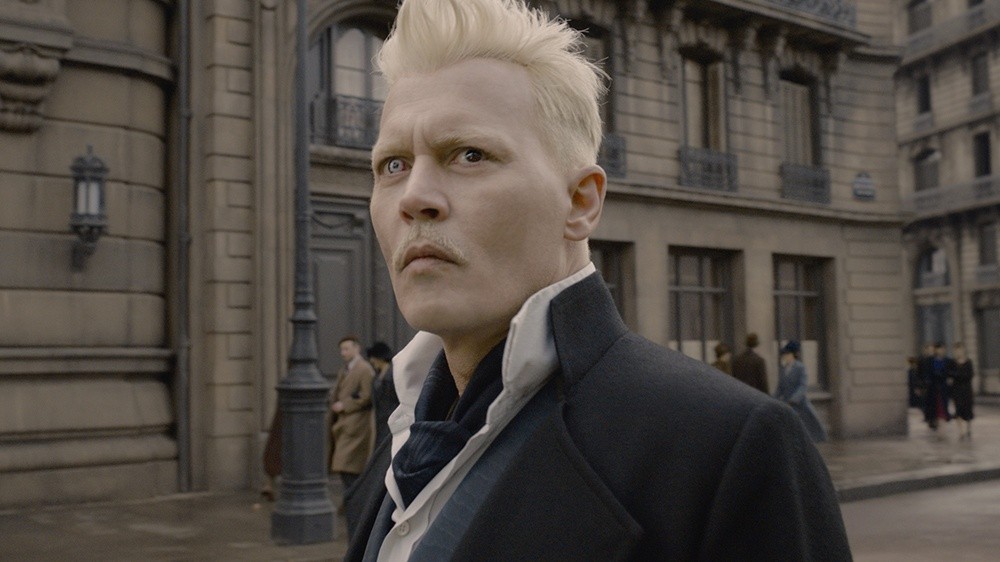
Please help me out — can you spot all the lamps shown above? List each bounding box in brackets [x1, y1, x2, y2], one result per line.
[70, 141, 110, 268]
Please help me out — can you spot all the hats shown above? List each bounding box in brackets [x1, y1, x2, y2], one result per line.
[366, 342, 392, 363]
[779, 341, 800, 357]
[714, 343, 730, 358]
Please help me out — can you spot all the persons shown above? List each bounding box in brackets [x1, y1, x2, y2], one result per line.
[775, 342, 826, 440]
[345, 1, 853, 562]
[733, 333, 768, 394]
[328, 337, 376, 488]
[712, 344, 733, 376]
[352, 342, 399, 450]
[909, 341, 974, 443]
